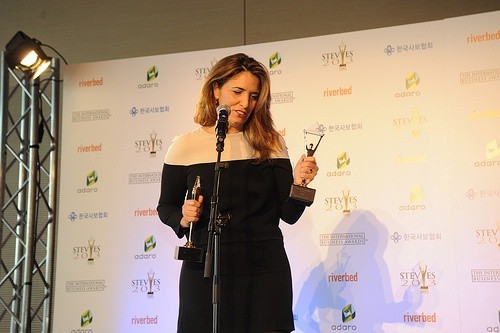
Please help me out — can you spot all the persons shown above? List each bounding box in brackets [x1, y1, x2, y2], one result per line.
[157, 52, 320, 333]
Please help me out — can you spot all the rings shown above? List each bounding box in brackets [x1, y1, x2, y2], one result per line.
[308, 168, 312, 174]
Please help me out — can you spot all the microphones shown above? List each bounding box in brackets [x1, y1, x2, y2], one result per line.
[216, 104, 231, 141]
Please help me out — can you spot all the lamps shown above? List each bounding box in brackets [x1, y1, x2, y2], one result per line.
[5, 31, 52, 80]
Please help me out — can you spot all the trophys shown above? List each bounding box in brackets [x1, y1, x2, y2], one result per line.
[173, 176, 203, 263]
[289, 129, 325, 207]
